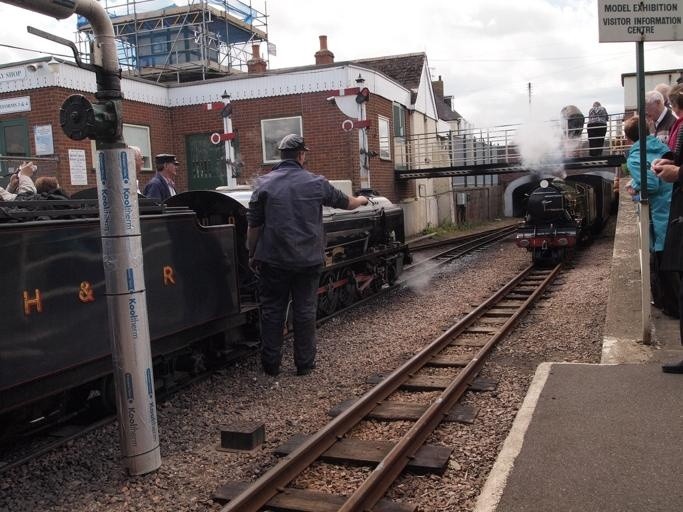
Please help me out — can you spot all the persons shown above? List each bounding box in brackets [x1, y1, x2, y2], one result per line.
[143, 154, 180, 204]
[35, 176, 60, 194]
[127, 145, 144, 194]
[246, 133, 368, 376]
[587, 102, 609, 157]
[623, 83, 682, 373]
[0, 160, 38, 202]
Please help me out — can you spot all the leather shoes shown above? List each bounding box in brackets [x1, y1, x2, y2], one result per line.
[297, 361, 317, 375]
[662, 359, 683, 374]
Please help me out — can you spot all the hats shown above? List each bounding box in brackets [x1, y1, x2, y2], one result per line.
[155, 154, 180, 164]
[277, 134, 310, 151]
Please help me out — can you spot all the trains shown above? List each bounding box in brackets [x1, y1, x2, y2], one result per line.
[512, 170, 616, 269]
[0, 176, 416, 448]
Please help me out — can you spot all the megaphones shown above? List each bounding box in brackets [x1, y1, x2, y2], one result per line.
[26, 63, 40, 73]
